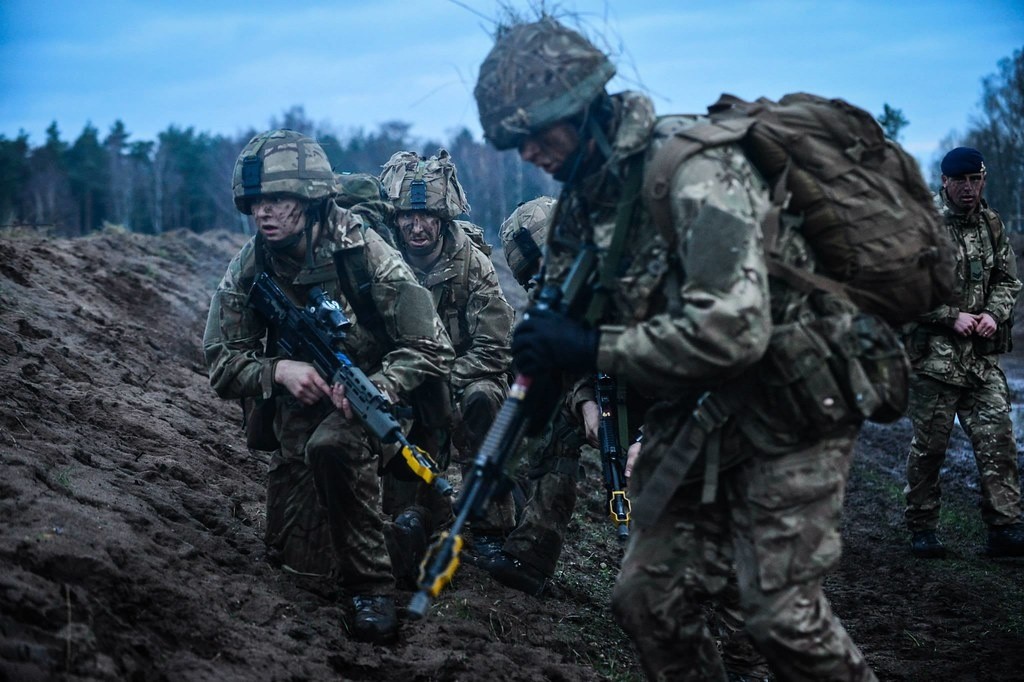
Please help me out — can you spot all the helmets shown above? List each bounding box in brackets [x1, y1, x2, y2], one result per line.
[500, 196, 557, 285]
[380, 151, 471, 222]
[232, 129, 338, 215]
[475, 17, 616, 152]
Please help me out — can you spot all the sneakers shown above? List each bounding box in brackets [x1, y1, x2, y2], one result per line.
[347, 595, 400, 644]
[391, 505, 433, 592]
[407, 492, 456, 526]
[471, 531, 502, 557]
[912, 529, 962, 559]
[988, 522, 1024, 558]
[475, 554, 546, 596]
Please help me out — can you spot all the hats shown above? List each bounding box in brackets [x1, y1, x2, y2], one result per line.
[941, 146, 986, 177]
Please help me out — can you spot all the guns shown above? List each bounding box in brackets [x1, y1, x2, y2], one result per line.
[247, 268, 461, 499]
[596, 371, 634, 543]
[404, 247, 608, 619]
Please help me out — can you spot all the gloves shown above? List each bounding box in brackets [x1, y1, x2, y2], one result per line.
[510, 308, 599, 379]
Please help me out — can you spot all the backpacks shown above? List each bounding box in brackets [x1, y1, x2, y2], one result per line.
[644, 93, 962, 327]
[243, 173, 398, 452]
[448, 219, 494, 350]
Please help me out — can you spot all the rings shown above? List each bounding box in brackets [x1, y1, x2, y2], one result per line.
[984, 332, 988, 336]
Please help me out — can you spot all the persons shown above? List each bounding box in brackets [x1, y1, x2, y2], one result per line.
[200, 129, 648, 642]
[474, 17, 877, 682]
[905, 147, 1024, 560]
